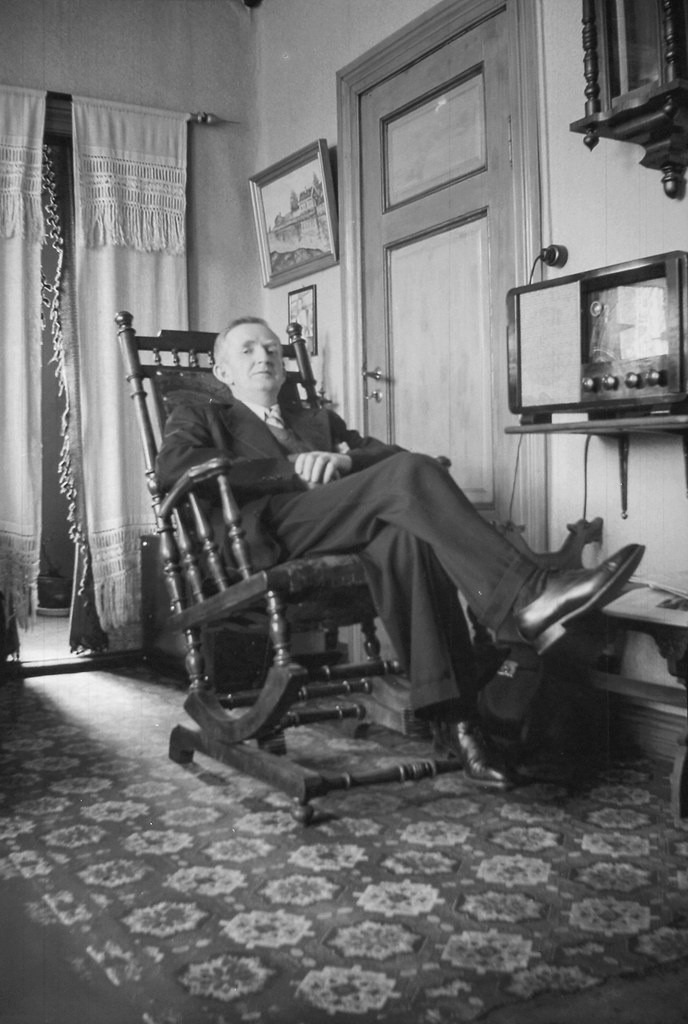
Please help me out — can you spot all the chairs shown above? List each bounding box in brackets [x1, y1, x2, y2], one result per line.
[113, 307, 460, 822]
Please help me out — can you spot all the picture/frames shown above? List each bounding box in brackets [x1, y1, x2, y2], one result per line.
[286, 282, 316, 354]
[246, 133, 338, 292]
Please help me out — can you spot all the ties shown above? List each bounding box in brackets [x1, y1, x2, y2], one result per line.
[265, 406, 287, 430]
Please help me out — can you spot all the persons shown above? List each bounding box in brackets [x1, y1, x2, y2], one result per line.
[155, 316, 645, 787]
[294, 298, 314, 353]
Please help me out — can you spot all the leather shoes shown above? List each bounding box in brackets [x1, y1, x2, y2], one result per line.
[511, 543, 645, 657]
[428, 706, 520, 789]
